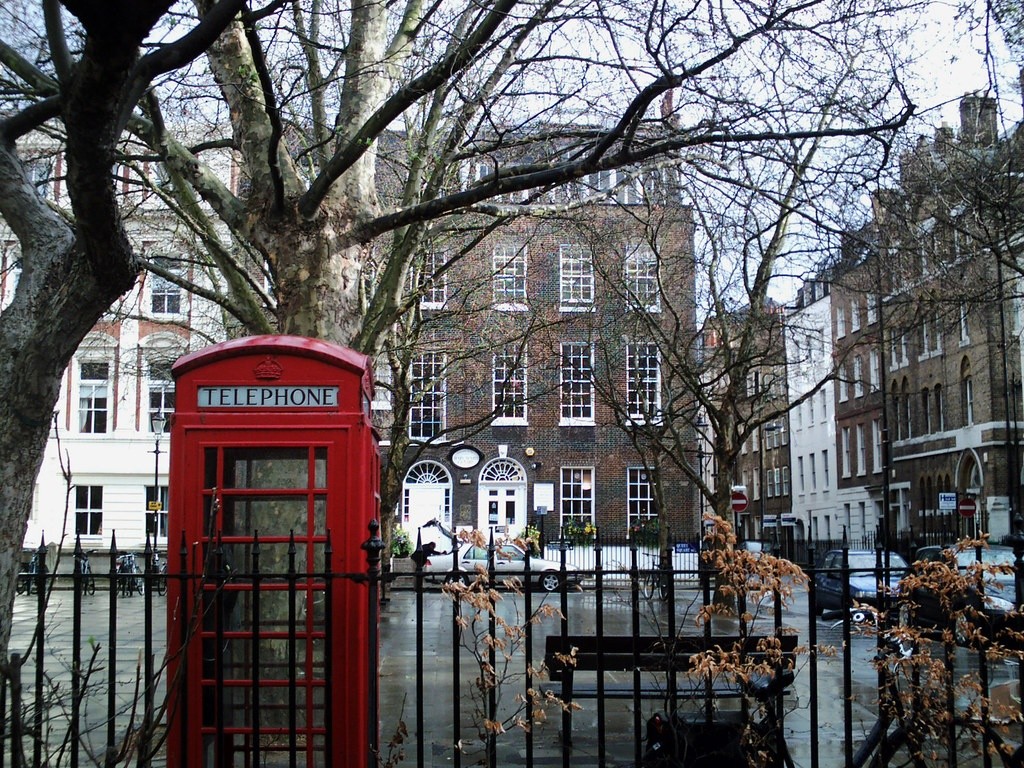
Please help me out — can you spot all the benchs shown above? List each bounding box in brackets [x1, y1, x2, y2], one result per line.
[538, 634, 799, 751]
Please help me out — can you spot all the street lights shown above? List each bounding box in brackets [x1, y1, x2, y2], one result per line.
[759, 425, 785, 586]
[144, 407, 168, 585]
[694, 412, 709, 585]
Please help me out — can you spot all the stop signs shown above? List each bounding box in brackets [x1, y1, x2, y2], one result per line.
[958, 498, 982, 517]
[730, 492, 751, 513]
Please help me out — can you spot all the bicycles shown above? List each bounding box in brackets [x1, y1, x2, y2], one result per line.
[72, 549, 98, 597]
[115, 551, 144, 599]
[644, 552, 669, 602]
[18, 546, 48, 595]
[157, 556, 169, 597]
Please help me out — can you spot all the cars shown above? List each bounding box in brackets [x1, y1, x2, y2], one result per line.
[813, 548, 910, 620]
[903, 545, 1016, 646]
[426, 539, 582, 594]
